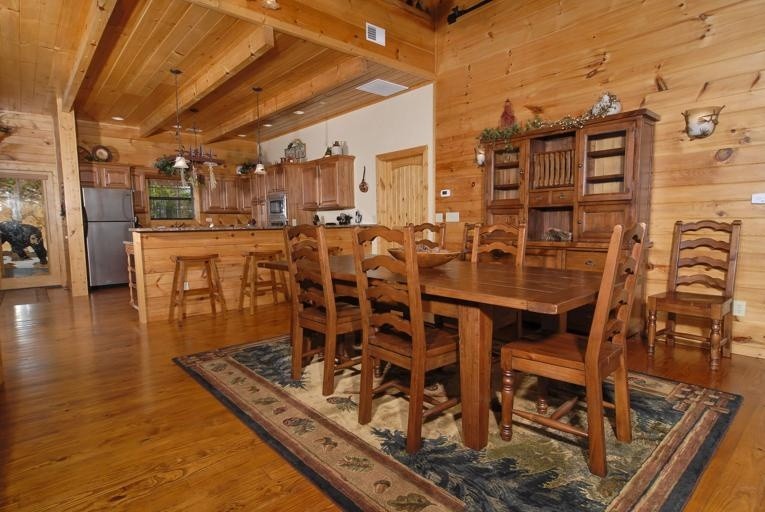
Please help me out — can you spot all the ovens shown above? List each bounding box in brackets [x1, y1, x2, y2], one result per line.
[267, 190, 289, 228]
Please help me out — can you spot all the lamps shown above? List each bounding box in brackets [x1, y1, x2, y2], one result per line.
[253, 87, 268, 178]
[171, 69, 189, 170]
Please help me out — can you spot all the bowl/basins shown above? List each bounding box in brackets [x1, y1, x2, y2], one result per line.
[387, 247, 462, 268]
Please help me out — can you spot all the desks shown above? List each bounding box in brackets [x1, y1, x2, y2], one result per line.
[132, 227, 372, 324]
[245, 253, 644, 448]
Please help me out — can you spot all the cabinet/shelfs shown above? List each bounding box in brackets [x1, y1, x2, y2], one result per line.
[480, 108, 661, 342]
[300, 156, 355, 211]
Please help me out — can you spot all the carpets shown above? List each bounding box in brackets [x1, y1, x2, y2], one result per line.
[171, 333, 744, 512]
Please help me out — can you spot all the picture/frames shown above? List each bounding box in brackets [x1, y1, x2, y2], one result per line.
[0, 173, 49, 278]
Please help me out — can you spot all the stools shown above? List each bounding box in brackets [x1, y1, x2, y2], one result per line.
[168, 253, 228, 328]
[124, 240, 137, 311]
[238, 249, 291, 315]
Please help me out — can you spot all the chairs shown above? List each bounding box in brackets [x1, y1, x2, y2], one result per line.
[442, 223, 528, 340]
[501, 220, 648, 478]
[646, 220, 742, 372]
[282, 223, 376, 397]
[349, 224, 499, 454]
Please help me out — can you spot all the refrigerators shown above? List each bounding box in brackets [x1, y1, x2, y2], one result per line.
[81, 187, 135, 291]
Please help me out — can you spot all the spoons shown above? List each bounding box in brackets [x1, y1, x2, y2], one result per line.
[359, 166, 368, 193]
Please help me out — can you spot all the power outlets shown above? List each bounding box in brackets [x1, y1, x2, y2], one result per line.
[752, 192, 765, 205]
[436, 212, 443, 223]
[445, 211, 461, 221]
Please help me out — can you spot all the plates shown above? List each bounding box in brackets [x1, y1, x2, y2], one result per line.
[92, 145, 112, 162]
